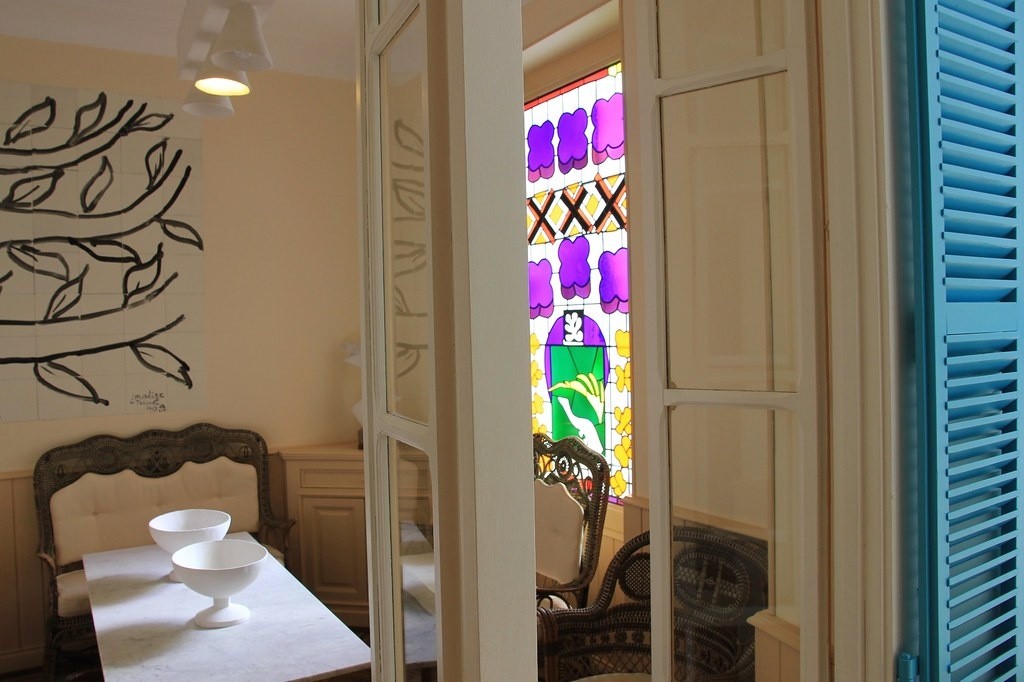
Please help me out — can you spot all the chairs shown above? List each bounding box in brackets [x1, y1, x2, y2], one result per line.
[536, 526, 768, 682]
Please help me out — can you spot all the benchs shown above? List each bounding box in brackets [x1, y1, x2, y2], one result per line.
[533, 432, 610, 677]
[32, 422, 298, 682]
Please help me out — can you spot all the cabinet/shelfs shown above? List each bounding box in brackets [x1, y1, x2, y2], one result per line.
[280, 443, 434, 627]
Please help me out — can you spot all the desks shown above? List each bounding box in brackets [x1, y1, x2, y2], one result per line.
[82, 532, 371, 682]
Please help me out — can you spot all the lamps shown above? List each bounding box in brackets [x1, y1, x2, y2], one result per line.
[177, 0, 276, 118]
